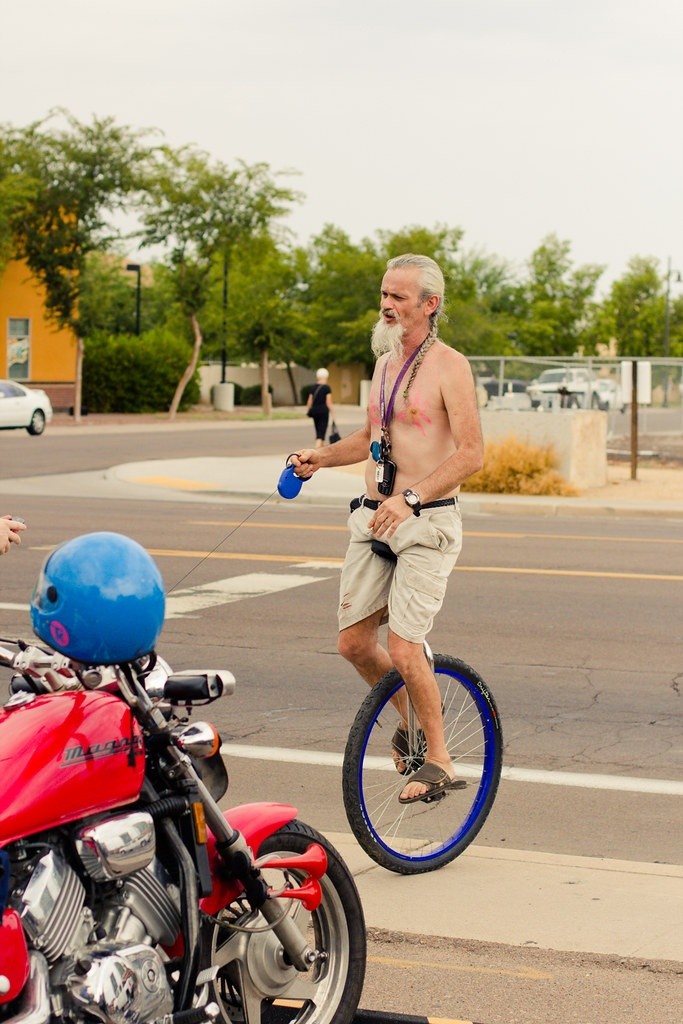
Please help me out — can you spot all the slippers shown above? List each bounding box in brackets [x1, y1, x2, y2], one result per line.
[391, 702, 445, 776]
[398, 762, 467, 804]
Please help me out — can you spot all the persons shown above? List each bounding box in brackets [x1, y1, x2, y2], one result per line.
[291, 253, 486, 803]
[527, 379, 543, 410]
[306, 368, 334, 448]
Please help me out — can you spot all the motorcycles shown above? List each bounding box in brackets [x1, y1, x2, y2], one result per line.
[0, 632, 372, 1024]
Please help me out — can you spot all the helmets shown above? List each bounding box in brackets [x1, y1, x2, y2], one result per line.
[30, 531, 164, 665]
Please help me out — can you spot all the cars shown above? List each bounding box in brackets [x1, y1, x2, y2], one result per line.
[475, 367, 623, 412]
[0, 378, 53, 436]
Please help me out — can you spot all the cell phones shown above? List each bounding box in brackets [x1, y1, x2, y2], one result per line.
[378, 460, 397, 495]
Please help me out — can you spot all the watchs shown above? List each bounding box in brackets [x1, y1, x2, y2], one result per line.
[402, 489, 421, 517]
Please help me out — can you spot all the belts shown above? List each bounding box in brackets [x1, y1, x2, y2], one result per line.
[360, 495, 458, 511]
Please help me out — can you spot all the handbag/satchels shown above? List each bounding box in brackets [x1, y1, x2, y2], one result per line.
[307, 408, 313, 417]
[329, 421, 341, 444]
